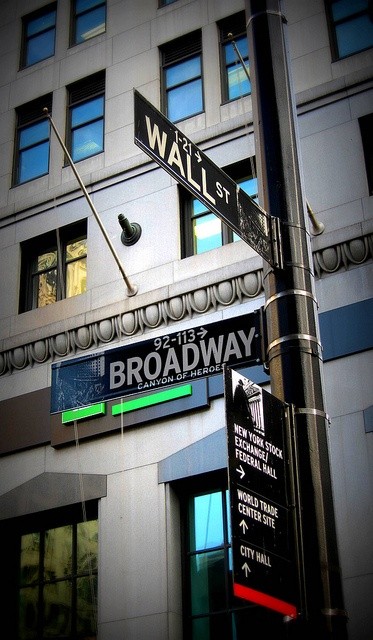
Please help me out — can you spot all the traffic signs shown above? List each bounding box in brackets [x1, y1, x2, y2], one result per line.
[223, 365, 295, 617]
[50, 309, 260, 416]
[133, 88, 272, 268]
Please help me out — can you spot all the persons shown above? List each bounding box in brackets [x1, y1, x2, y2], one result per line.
[234, 379, 255, 422]
